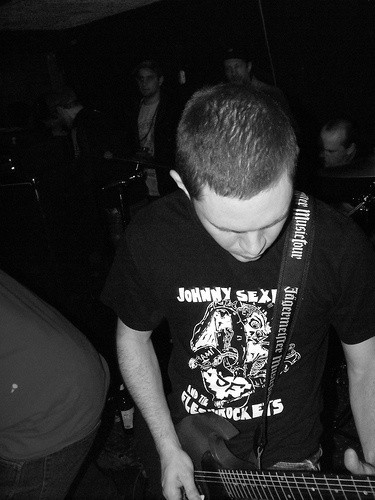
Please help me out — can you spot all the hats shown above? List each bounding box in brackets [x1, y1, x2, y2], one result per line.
[222, 46, 248, 60]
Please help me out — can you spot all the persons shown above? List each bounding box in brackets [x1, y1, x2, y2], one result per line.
[102, 83, 375, 500]
[37, 87, 114, 207]
[0, 269, 112, 500]
[320, 117, 358, 169]
[223, 47, 292, 121]
[116, 61, 181, 203]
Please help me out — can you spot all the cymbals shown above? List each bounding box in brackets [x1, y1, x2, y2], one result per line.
[111, 150, 158, 168]
[315, 159, 375, 177]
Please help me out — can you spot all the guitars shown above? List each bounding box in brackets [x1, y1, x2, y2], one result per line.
[133, 389, 375, 500]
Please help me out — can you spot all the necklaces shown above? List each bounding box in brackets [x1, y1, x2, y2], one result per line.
[137, 100, 160, 142]
